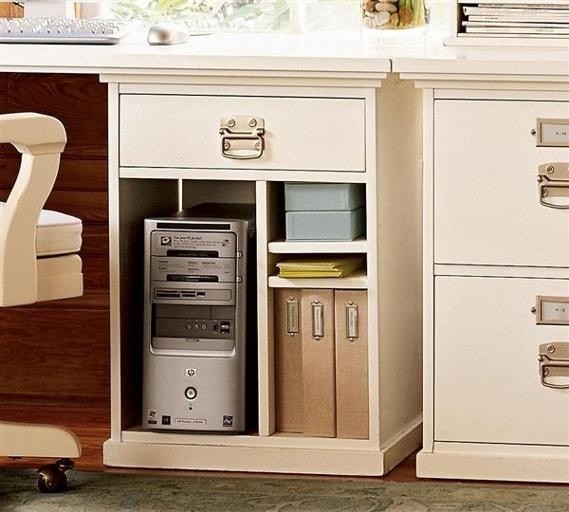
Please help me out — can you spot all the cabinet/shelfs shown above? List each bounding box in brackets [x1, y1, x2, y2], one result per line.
[98, 69, 423, 478]
[398, 72, 569, 485]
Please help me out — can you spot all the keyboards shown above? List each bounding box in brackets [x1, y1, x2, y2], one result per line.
[0, 16, 133, 46]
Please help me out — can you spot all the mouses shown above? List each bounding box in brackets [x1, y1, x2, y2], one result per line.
[146, 25, 175, 45]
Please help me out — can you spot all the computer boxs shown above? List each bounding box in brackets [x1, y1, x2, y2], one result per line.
[142, 202, 256, 433]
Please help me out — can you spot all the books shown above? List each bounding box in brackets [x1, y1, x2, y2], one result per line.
[457, 4, 569, 38]
[275, 254, 366, 280]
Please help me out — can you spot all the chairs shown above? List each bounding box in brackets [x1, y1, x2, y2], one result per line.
[0, 110, 87, 495]
